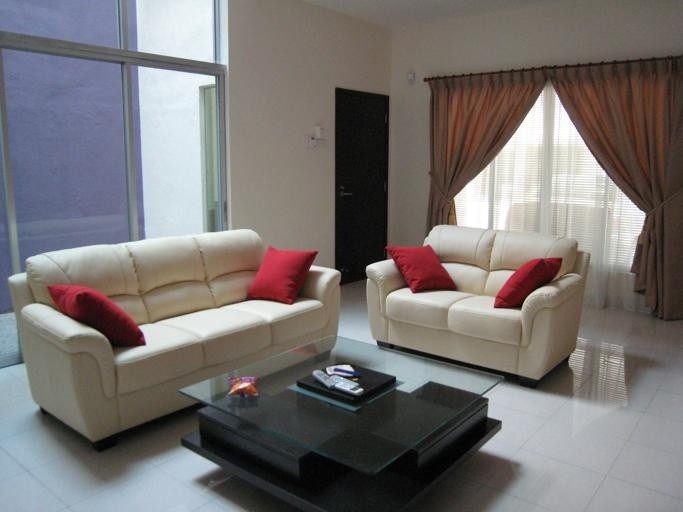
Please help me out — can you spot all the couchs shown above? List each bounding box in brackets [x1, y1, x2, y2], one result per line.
[363, 224, 590, 389]
[7, 227, 342, 453]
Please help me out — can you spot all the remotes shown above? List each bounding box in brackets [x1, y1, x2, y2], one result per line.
[330, 374, 365, 397]
[312, 369, 335, 389]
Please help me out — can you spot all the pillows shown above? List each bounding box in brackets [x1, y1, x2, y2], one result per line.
[384, 239, 460, 294]
[493, 256, 562, 308]
[46, 283, 147, 347]
[244, 244, 318, 306]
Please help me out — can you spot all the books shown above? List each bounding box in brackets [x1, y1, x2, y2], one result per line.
[295, 361, 397, 406]
